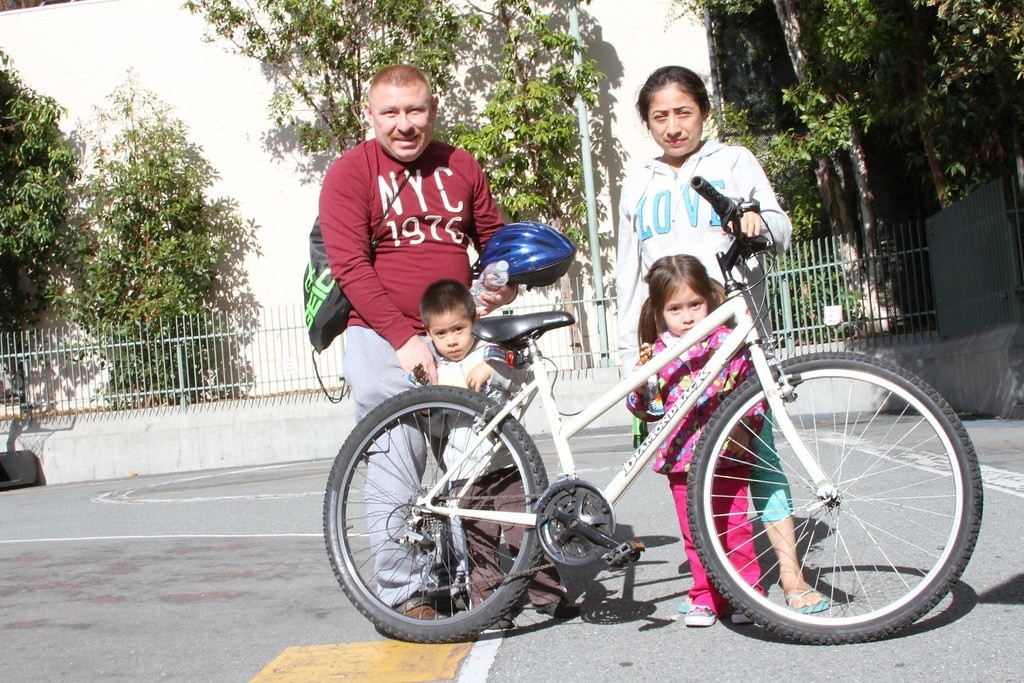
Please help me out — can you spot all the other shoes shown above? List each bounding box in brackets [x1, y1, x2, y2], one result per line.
[489, 611, 513, 630]
[394, 596, 448, 621]
[537, 597, 580, 619]
[446, 592, 469, 611]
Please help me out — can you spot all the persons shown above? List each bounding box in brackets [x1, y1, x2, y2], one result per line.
[320, 65, 519, 621]
[626, 256, 769, 627]
[420, 281, 586, 628]
[615, 66, 830, 614]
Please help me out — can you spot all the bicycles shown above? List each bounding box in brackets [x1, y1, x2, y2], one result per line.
[321, 176, 986, 647]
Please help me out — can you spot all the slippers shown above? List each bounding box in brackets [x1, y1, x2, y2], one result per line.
[678, 598, 693, 613]
[786, 584, 829, 614]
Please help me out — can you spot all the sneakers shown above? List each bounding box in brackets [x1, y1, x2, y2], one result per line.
[731, 607, 754, 624]
[685, 605, 718, 626]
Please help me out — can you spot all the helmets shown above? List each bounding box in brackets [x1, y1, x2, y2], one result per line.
[471, 222, 576, 292]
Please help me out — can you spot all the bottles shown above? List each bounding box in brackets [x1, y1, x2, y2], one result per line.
[469, 260, 508, 315]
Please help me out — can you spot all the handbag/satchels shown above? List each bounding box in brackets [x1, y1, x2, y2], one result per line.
[303, 215, 379, 356]
